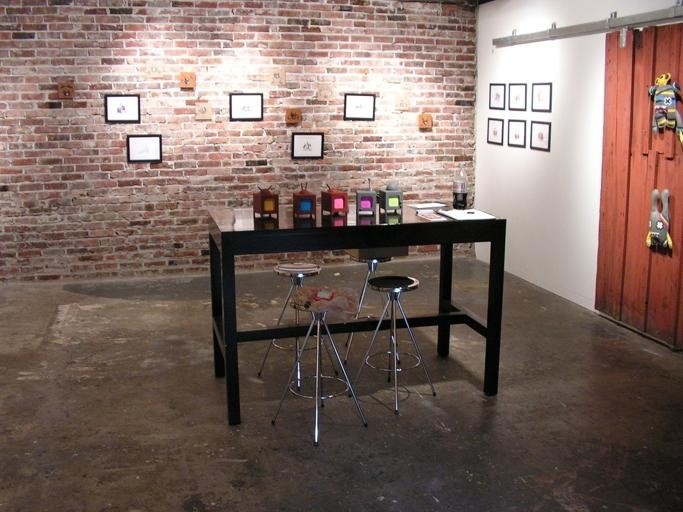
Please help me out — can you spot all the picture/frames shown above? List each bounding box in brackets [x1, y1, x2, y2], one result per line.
[508, 120, 526, 147]
[292, 132, 324, 159]
[229, 93, 263, 121]
[509, 83, 526, 110]
[127, 134, 162, 162]
[532, 83, 552, 112]
[530, 122, 551, 152]
[104, 94, 140, 124]
[489, 83, 505, 110]
[487, 118, 504, 145]
[344, 93, 375, 120]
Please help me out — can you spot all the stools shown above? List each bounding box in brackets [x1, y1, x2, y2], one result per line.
[257, 256, 435, 447]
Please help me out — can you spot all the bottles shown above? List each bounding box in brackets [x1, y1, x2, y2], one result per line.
[452, 162, 468, 210]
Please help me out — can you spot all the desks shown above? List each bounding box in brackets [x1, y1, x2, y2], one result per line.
[208, 201, 507, 426]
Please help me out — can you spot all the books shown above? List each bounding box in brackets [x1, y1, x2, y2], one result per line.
[415, 208, 448, 221]
[407, 202, 447, 208]
[435, 206, 496, 221]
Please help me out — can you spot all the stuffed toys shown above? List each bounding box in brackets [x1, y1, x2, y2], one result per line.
[645, 188, 673, 254]
[645, 73, 683, 145]
